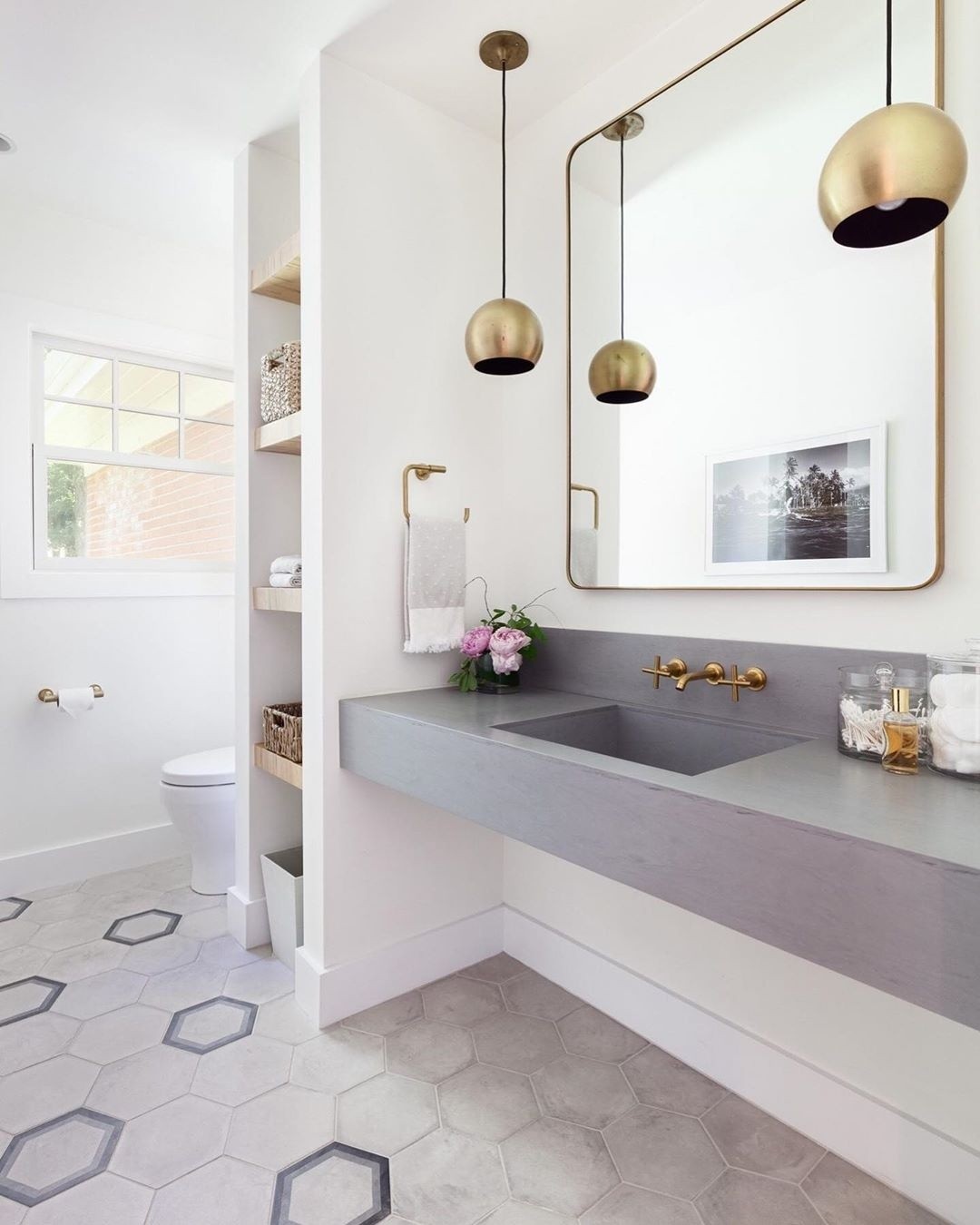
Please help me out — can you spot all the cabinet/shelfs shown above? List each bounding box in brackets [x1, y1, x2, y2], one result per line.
[224, 82, 321, 1026]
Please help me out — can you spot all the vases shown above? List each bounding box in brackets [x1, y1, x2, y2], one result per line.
[474, 654, 518, 694]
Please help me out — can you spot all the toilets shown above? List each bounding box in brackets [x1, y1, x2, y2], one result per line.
[158, 743, 238, 894]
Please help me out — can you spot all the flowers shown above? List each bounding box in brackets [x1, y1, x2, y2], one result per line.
[447, 574, 561, 700]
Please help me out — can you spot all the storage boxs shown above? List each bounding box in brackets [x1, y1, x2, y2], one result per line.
[262, 703, 302, 761]
[260, 342, 300, 420]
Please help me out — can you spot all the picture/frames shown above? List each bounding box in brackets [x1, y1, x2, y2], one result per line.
[705, 421, 889, 574]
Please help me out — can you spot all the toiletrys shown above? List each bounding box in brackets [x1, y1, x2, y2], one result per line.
[880, 687, 920, 776]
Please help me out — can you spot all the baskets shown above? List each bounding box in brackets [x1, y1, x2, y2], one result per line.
[260, 341, 301, 423]
[261, 701, 303, 763]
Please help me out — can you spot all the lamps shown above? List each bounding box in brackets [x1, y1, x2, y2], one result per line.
[821, 0, 966, 248]
[588, 113, 656, 404]
[462, 32, 542, 376]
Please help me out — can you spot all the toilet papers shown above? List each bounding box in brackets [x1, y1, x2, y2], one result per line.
[58, 685, 95, 715]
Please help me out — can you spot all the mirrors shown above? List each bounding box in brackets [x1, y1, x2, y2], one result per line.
[561, 0, 944, 591]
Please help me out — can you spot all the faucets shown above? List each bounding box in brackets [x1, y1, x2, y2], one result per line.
[675, 662, 724, 692]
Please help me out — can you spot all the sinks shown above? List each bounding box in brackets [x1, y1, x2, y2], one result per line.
[489, 703, 820, 780]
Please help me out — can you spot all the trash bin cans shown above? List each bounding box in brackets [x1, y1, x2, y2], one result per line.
[261, 844, 302, 970]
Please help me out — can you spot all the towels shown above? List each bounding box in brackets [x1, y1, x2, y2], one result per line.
[269, 557, 303, 587]
[400, 510, 467, 653]
